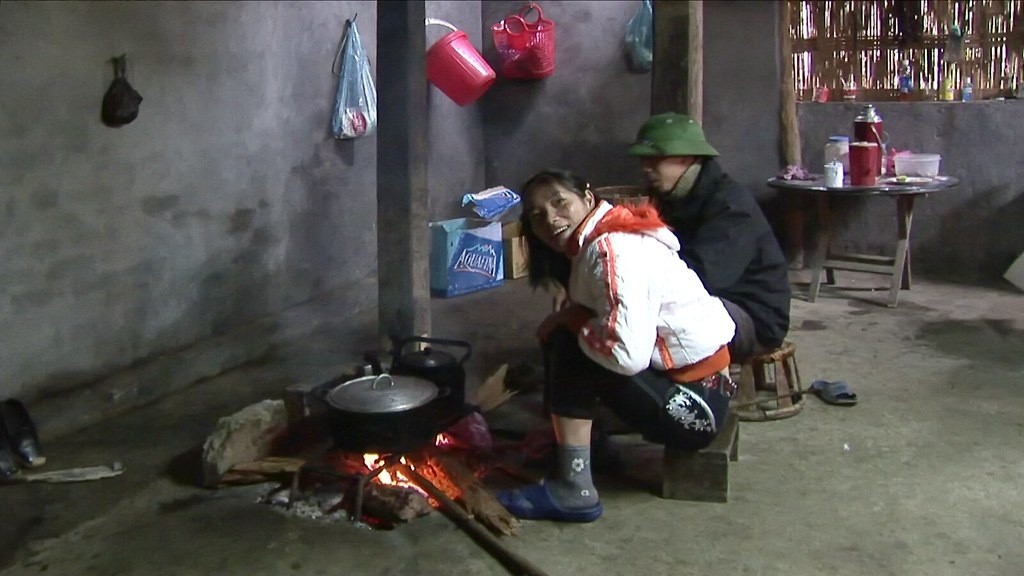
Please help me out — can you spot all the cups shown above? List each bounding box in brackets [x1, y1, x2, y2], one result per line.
[811, 86, 829, 103]
[821, 162, 845, 189]
[843, 89, 858, 100]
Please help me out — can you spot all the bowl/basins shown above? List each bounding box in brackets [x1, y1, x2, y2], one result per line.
[893, 153, 942, 177]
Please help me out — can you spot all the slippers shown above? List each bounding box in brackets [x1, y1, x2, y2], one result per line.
[498, 477, 602, 523]
[522, 444, 622, 468]
[812, 380, 858, 404]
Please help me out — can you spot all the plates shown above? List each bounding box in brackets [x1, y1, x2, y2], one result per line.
[887, 177, 933, 185]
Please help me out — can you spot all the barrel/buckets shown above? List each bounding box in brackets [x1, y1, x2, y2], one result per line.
[424, 17, 497, 107]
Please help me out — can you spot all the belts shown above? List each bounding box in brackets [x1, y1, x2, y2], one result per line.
[690, 372, 734, 398]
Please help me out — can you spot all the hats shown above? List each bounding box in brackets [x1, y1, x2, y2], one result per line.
[624, 114, 720, 157]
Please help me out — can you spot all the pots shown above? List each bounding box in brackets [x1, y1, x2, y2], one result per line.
[313, 373, 455, 447]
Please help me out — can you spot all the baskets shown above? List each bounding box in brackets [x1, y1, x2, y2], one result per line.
[490, 4, 555, 77]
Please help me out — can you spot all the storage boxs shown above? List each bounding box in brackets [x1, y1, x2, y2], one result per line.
[501, 221, 530, 279]
[428, 217, 505, 297]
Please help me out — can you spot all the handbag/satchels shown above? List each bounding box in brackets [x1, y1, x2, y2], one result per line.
[331, 23, 378, 139]
[106, 56, 142, 122]
[624, 0, 653, 73]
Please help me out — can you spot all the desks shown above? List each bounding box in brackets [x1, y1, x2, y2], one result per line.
[769, 171, 960, 308]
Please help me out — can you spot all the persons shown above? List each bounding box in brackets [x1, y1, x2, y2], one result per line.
[498, 172, 737, 523]
[627, 112, 791, 359]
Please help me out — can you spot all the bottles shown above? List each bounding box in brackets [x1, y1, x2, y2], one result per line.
[823, 134, 851, 173]
[962, 76, 973, 101]
[881, 144, 886, 176]
[899, 59, 913, 101]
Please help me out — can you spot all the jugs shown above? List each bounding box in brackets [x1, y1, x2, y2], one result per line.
[851, 103, 889, 180]
[849, 141, 878, 186]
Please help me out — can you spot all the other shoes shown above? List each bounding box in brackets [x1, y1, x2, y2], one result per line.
[0, 398, 46, 480]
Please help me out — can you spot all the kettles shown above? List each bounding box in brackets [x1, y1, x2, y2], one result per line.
[360, 335, 472, 409]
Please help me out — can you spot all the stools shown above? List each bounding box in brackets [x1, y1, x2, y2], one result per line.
[729, 339, 806, 423]
[664, 413, 740, 502]
